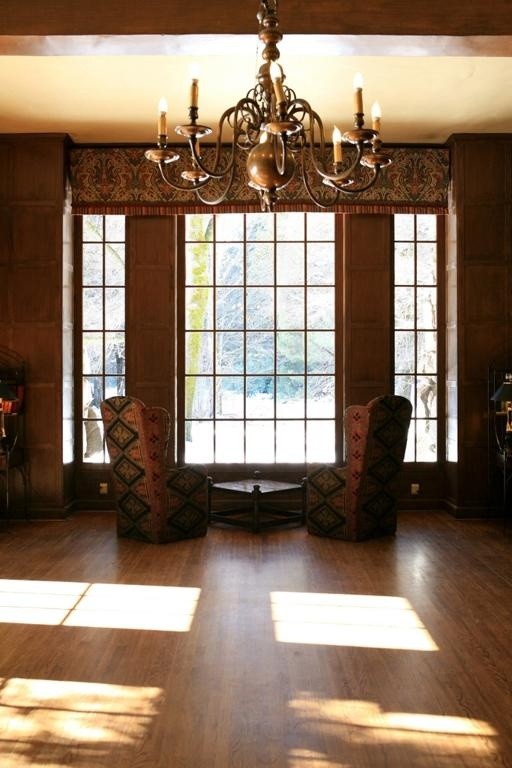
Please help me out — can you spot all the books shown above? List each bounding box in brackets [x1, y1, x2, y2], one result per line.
[0, 398, 21, 414]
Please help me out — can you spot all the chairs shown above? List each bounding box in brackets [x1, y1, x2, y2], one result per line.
[306, 394, 412, 543]
[100, 396, 208, 545]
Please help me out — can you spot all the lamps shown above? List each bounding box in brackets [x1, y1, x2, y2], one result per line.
[145, 0, 395, 213]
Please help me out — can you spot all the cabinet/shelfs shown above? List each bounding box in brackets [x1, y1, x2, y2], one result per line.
[0, 344, 32, 524]
[487, 352, 512, 530]
